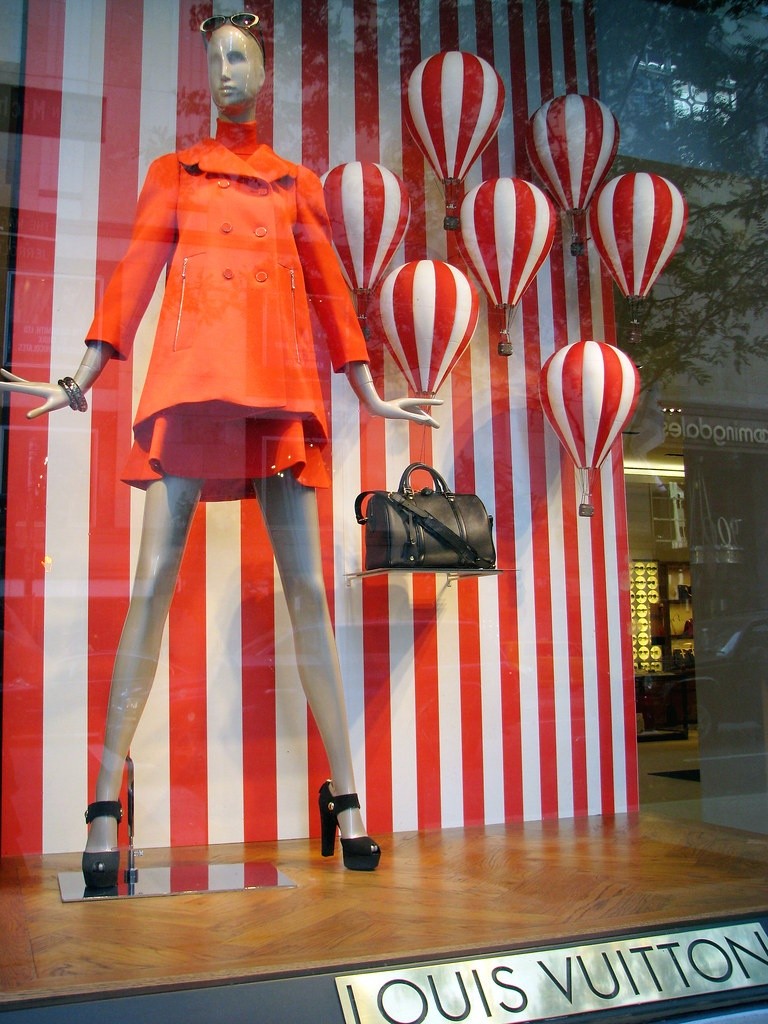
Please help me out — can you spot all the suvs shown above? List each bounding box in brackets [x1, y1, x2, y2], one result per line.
[641, 616, 768, 727]
[104, 619, 504, 760]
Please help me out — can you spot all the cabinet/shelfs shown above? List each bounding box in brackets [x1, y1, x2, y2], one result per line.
[650, 559, 694, 670]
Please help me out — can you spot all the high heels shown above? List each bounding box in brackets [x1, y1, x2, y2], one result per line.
[319, 779, 381, 870]
[82, 798, 123, 887]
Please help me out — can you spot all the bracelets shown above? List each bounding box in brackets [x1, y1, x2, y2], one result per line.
[57, 376, 88, 413]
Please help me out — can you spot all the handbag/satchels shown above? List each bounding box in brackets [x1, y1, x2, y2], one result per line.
[678, 585, 692, 599]
[683, 619, 693, 636]
[355, 462, 496, 570]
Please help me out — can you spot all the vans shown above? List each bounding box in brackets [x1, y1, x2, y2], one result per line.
[222, 622, 431, 669]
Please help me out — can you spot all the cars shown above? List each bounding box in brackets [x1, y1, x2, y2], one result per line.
[643, 677, 720, 728]
[3, 647, 185, 720]
[635, 672, 683, 697]
[528, 640, 584, 676]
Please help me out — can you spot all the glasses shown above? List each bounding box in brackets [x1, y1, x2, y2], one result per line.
[631, 581, 660, 669]
[635, 567, 643, 571]
[200, 12, 265, 69]
[647, 567, 657, 571]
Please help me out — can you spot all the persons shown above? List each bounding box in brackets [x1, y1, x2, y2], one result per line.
[0, 14, 443, 891]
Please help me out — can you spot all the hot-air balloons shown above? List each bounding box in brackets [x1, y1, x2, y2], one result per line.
[318, 48, 688, 516]
